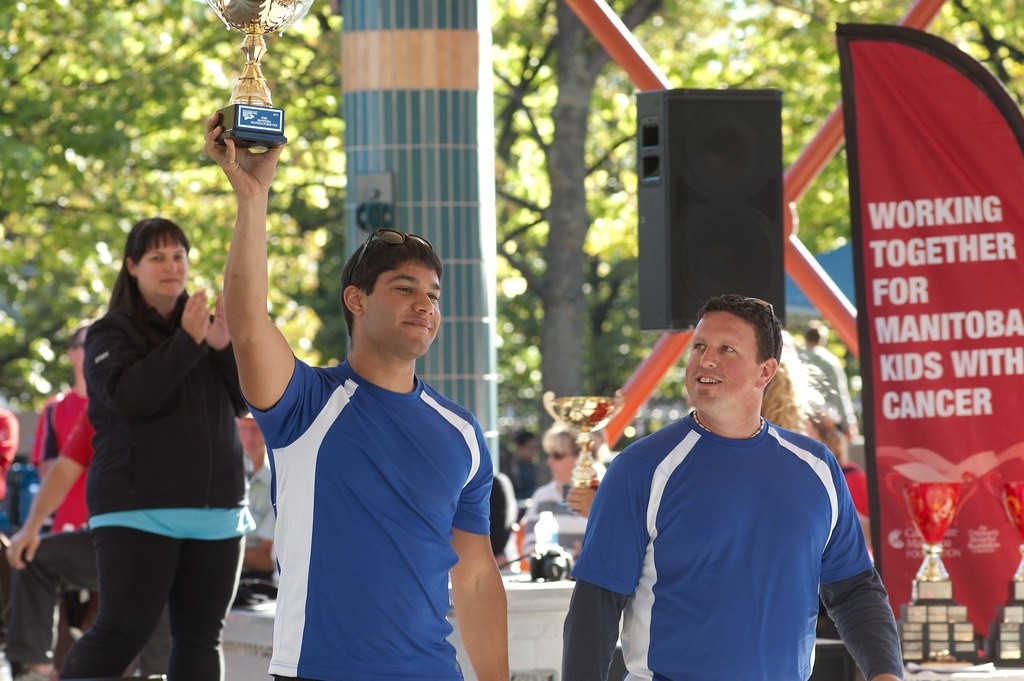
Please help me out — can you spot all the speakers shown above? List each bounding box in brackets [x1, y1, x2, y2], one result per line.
[635, 88, 787, 330]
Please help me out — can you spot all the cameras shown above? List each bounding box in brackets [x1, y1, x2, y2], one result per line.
[531, 545, 575, 583]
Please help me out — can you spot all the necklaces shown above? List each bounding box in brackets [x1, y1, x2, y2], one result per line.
[693, 411, 764, 438]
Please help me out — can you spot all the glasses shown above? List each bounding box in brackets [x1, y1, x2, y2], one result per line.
[353, 229, 433, 270]
[722, 294, 775, 332]
[554, 452, 568, 460]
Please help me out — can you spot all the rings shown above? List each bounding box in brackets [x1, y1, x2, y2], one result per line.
[224, 160, 236, 164]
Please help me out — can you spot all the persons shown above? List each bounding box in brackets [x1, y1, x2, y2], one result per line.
[0, 217, 279, 681]
[490, 429, 605, 573]
[205, 111, 509, 681]
[760, 321, 872, 637]
[562, 297, 902, 681]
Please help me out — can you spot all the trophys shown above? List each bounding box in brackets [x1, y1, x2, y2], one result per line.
[983, 472, 1024, 667]
[886, 471, 980, 664]
[205, 0, 314, 148]
[543, 390, 625, 512]
